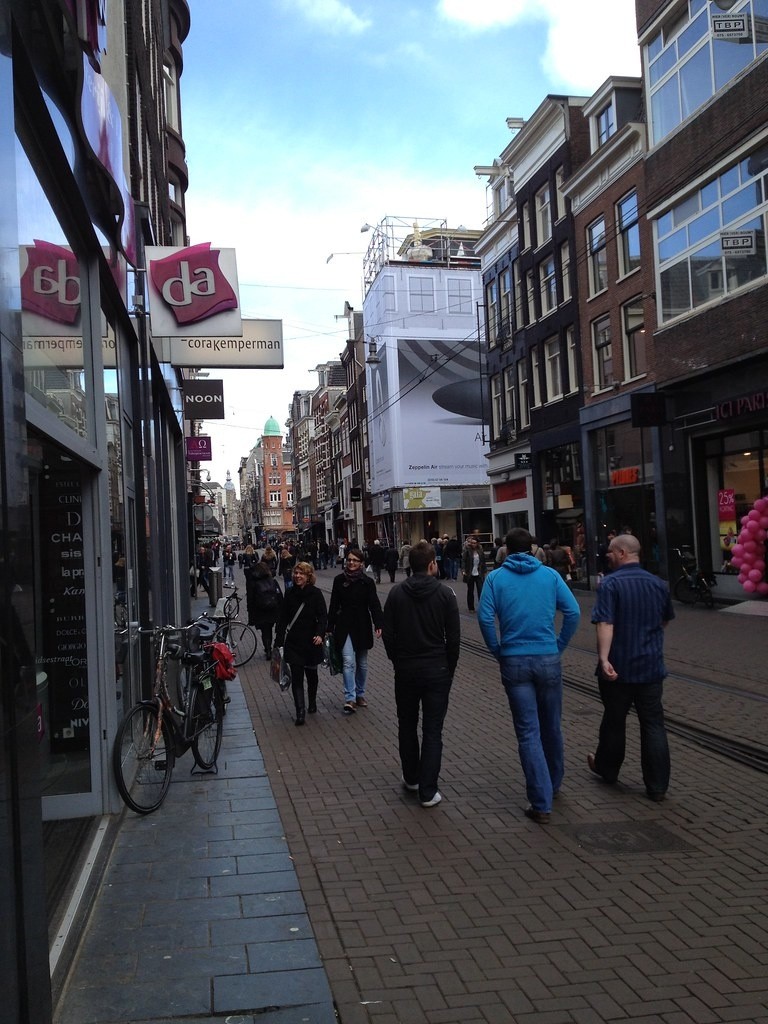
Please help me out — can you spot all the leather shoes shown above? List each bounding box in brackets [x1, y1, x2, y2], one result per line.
[587, 753, 617, 784]
[646, 789, 665, 801]
[356, 697, 368, 706]
[344, 701, 357, 713]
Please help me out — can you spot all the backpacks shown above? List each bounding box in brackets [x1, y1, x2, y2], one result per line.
[259, 591, 278, 611]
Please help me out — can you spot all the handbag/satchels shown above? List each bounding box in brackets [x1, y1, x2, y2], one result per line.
[365, 565, 373, 573]
[479, 563, 487, 573]
[270, 646, 291, 692]
[321, 635, 343, 676]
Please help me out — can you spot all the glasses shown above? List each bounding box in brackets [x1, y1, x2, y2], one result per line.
[347, 558, 360, 562]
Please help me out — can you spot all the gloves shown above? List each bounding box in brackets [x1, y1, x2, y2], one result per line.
[273, 632, 287, 652]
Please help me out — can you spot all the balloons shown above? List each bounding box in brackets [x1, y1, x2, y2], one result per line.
[730, 497, 768, 594]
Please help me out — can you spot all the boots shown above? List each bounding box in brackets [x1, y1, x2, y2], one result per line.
[292, 687, 306, 726]
[307, 687, 317, 713]
[264, 639, 272, 660]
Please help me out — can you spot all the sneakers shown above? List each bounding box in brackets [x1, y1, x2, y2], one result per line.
[421, 792, 441, 807]
[402, 774, 419, 789]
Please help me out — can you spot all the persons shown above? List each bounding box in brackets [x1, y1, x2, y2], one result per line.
[477, 527, 581, 824]
[197, 533, 576, 614]
[326, 549, 384, 713]
[381, 541, 462, 808]
[246, 563, 283, 660]
[274, 562, 328, 728]
[585, 526, 679, 800]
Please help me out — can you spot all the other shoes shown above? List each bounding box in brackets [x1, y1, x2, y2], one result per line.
[551, 788, 560, 798]
[470, 610, 474, 613]
[524, 804, 550, 823]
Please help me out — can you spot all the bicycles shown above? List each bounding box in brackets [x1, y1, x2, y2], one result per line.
[112, 611, 231, 814]
[672, 548, 714, 609]
[210, 585, 257, 668]
